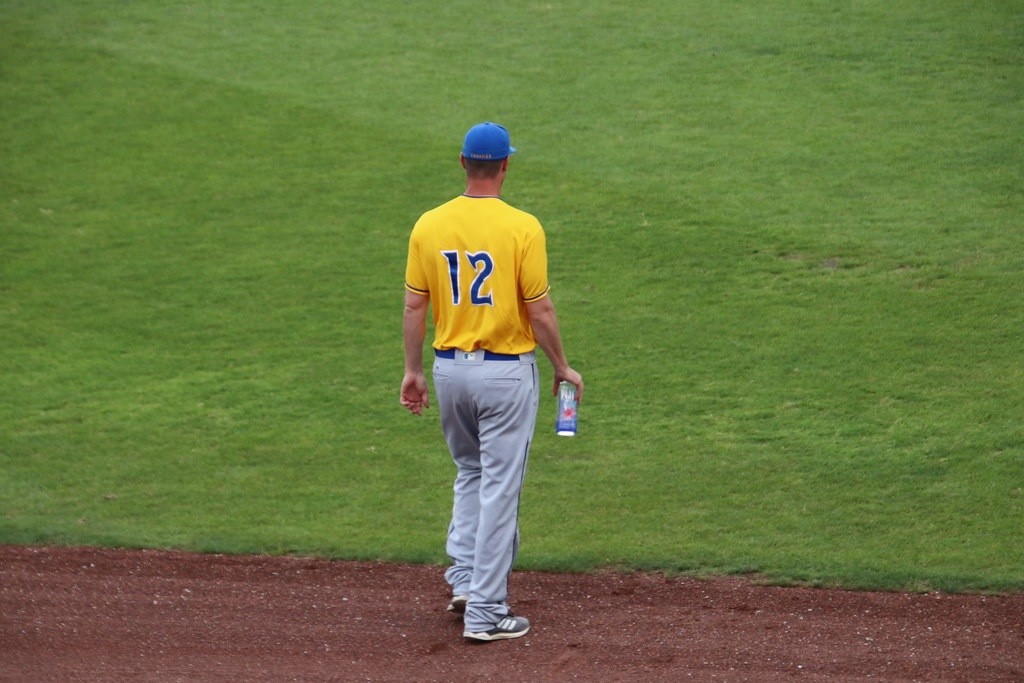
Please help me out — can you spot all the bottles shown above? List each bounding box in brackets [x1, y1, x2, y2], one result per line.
[555, 381, 579, 437]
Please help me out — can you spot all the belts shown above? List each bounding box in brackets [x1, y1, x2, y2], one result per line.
[435, 348, 519, 361]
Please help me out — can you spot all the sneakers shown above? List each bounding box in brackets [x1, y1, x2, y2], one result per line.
[445, 594, 468, 613]
[463, 615, 529, 640]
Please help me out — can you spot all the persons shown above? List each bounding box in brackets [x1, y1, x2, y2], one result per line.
[399, 124, 583, 642]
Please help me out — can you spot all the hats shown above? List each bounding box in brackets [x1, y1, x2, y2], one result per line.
[462, 122, 516, 160]
